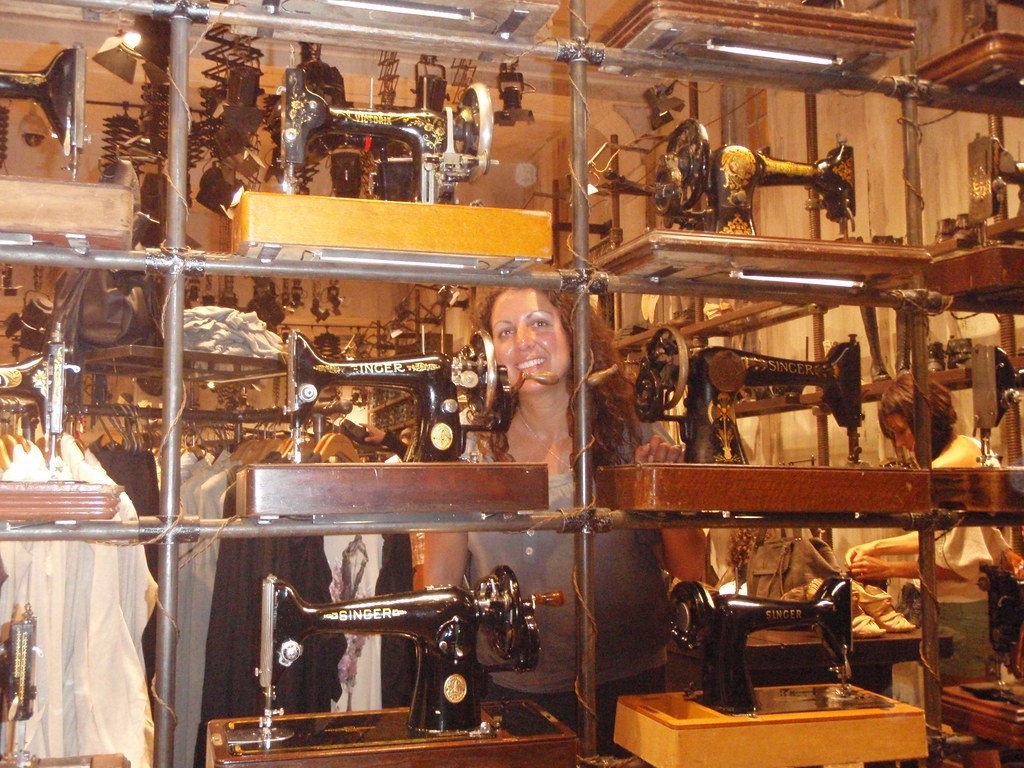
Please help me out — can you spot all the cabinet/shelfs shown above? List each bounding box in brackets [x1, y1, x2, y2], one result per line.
[0, 0, 1024, 768]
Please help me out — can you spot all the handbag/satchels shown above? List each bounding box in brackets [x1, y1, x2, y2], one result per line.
[746, 537, 842, 600]
[52, 268, 164, 348]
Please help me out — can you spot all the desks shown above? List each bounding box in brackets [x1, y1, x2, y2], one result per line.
[667, 625, 954, 700]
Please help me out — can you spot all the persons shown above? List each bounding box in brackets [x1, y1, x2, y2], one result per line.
[359, 272, 707, 768]
[847, 375, 1024, 768]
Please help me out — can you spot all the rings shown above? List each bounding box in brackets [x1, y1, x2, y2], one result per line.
[861, 568, 863, 575]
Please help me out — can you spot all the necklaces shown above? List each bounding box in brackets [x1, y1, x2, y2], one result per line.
[516, 409, 573, 472]
